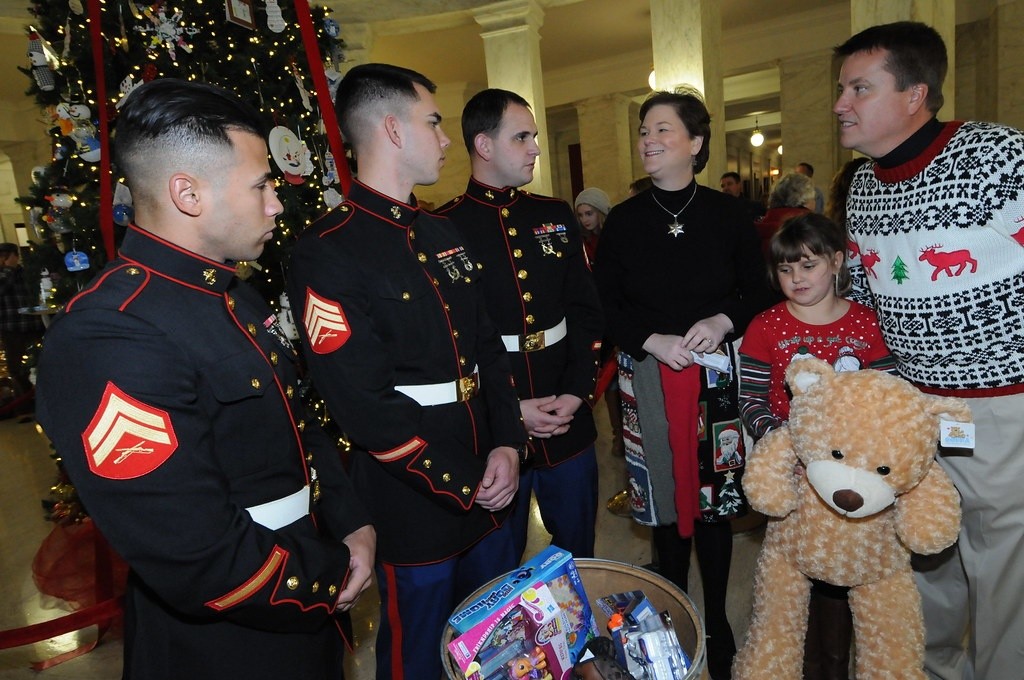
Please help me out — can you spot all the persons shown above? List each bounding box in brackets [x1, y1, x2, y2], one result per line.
[50, 265, 66, 304]
[598, 91, 767, 680]
[720, 172, 764, 214]
[627, 175, 653, 198]
[830, 157, 871, 237]
[832, 19, 1024, 680]
[293, 63, 526, 680]
[0, 242, 40, 395]
[36, 79, 378, 680]
[736, 216, 895, 680]
[795, 162, 823, 215]
[754, 173, 816, 266]
[432, 88, 594, 568]
[575, 188, 625, 457]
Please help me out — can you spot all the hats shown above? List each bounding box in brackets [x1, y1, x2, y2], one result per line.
[575, 188, 610, 215]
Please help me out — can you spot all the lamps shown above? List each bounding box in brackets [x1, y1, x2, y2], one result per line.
[750, 116, 765, 147]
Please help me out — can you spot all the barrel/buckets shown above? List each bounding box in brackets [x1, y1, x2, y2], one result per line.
[454, 569, 708, 680]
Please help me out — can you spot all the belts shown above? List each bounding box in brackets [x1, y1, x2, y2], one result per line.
[245, 468, 320, 530]
[394, 364, 481, 407]
[501, 317, 567, 352]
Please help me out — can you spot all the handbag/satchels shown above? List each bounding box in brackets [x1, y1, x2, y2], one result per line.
[803, 589, 851, 680]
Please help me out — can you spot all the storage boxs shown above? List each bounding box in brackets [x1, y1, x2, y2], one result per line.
[447, 544, 599, 679]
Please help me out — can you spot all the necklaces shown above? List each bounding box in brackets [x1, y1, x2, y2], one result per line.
[650, 183, 698, 238]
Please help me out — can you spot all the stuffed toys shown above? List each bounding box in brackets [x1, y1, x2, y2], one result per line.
[730, 359, 972, 680]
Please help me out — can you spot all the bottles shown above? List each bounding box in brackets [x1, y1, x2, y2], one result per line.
[40, 271, 52, 302]
